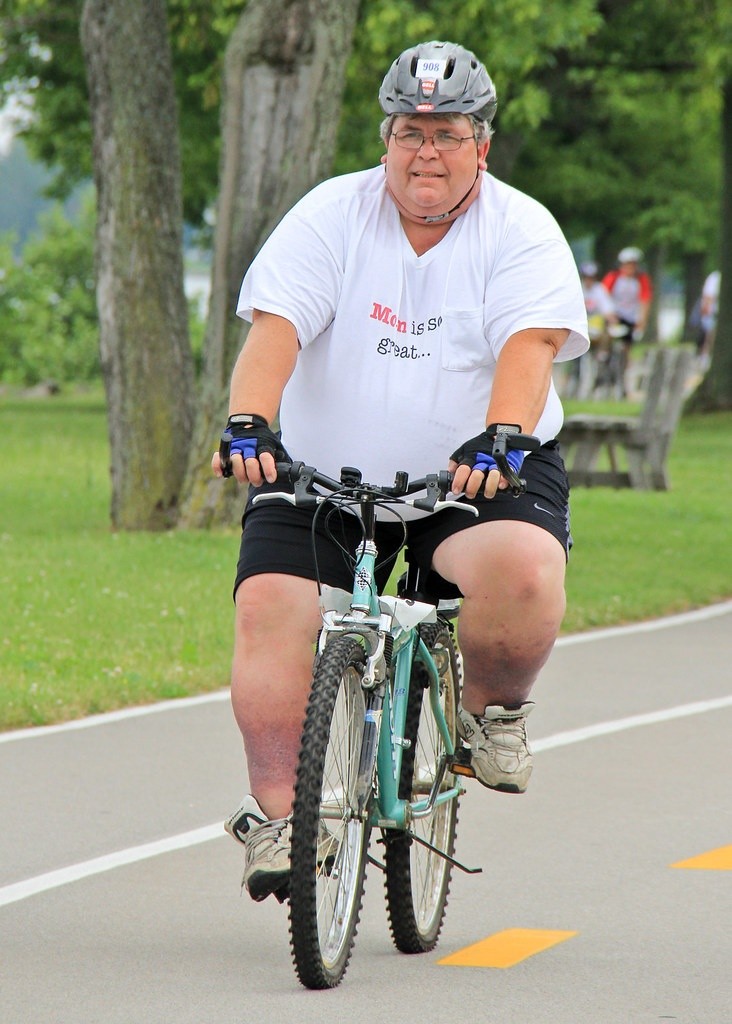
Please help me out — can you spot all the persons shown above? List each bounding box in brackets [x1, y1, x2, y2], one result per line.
[210, 36, 591, 898]
[581, 243, 722, 391]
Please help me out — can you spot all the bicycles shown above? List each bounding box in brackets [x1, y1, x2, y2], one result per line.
[209, 451, 526, 992]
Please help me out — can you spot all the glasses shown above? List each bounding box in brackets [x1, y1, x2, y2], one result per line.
[388, 129, 476, 151]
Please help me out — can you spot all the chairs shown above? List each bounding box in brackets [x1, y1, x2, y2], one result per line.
[554, 338, 694, 494]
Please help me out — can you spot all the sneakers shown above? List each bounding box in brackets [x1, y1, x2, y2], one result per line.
[456, 700, 536, 793]
[224, 794, 340, 901]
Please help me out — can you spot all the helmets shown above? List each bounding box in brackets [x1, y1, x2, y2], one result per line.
[379, 40, 497, 127]
